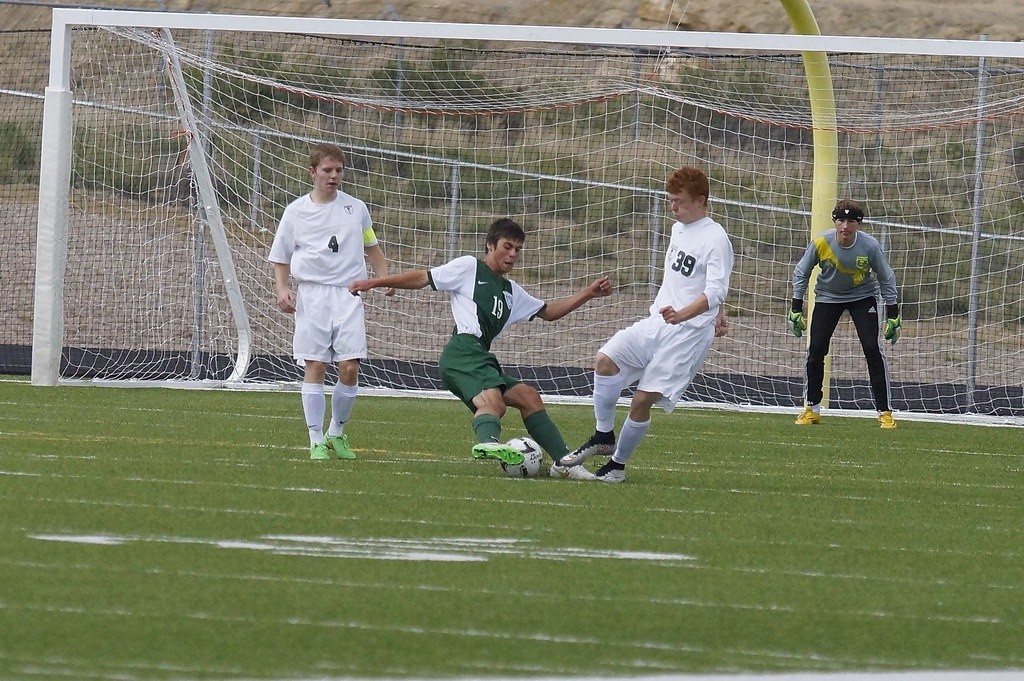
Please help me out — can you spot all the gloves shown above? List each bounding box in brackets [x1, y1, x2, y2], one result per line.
[786, 308, 806, 338]
[884, 314, 902, 346]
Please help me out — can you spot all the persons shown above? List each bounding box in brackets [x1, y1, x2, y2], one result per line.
[788, 201, 902, 428]
[267, 143, 396, 460]
[348, 218, 614, 480]
[560, 166, 735, 482]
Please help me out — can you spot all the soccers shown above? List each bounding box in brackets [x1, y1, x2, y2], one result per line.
[499, 434, 543, 478]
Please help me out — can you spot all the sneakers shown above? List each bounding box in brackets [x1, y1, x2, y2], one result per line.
[795, 406, 820, 425]
[324, 432, 356, 460]
[549, 461, 594, 480]
[877, 411, 901, 428]
[309, 442, 329, 459]
[560, 436, 615, 466]
[472, 436, 525, 465]
[594, 469, 625, 484]
